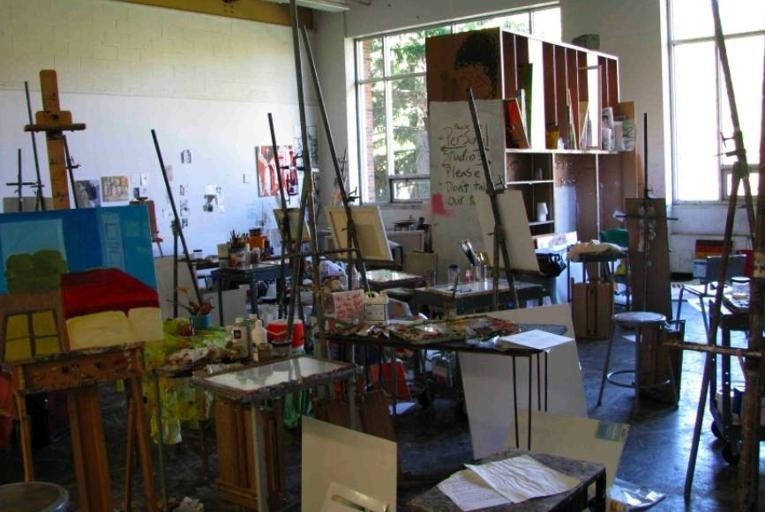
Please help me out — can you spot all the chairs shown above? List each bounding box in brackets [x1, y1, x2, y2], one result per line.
[678, 254, 746, 343]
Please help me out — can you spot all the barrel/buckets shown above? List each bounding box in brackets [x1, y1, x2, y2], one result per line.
[363, 289, 392, 327]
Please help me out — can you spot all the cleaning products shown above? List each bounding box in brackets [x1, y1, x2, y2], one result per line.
[231, 312, 267, 363]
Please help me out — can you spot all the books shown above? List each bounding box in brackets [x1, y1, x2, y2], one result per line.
[503, 98, 531, 149]
[516, 89, 529, 137]
[564, 86, 636, 156]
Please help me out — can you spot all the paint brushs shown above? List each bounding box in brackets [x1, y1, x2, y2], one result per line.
[459, 239, 480, 265]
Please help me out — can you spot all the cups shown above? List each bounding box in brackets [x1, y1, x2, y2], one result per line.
[192, 312, 212, 333]
[447, 264, 487, 291]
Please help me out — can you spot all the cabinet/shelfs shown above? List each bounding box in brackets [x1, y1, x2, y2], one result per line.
[425, 27, 643, 264]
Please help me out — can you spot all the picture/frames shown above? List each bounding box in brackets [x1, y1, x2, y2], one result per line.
[272, 207, 311, 244]
[327, 206, 394, 262]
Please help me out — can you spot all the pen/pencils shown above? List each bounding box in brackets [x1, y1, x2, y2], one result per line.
[229, 229, 248, 248]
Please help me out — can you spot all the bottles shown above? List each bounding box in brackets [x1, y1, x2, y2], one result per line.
[193, 249, 203, 265]
[251, 320, 268, 361]
[232, 313, 259, 358]
[251, 247, 261, 262]
[731, 277, 751, 305]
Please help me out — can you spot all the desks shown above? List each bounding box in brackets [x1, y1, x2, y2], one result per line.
[565, 244, 631, 337]
[413, 278, 544, 318]
[314, 323, 563, 450]
[212, 255, 325, 325]
[706, 298, 764, 464]
[9, 342, 158, 511]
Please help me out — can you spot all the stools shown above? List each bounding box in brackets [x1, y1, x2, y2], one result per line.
[597, 312, 679, 415]
[403, 448, 568, 511]
[201, 355, 365, 511]
